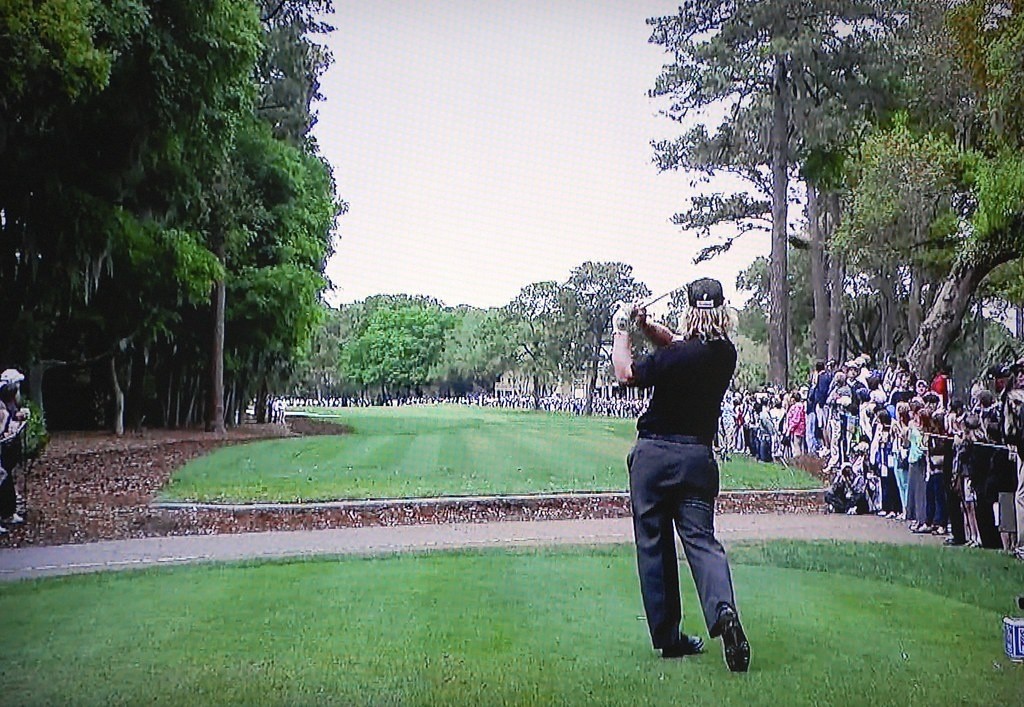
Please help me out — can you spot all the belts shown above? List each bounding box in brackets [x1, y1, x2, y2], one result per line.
[646, 433, 704, 444]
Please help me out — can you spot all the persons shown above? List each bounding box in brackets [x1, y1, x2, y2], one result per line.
[611, 278, 750, 672]
[712, 354, 1024, 559]
[267, 395, 650, 425]
[0, 369, 30, 534]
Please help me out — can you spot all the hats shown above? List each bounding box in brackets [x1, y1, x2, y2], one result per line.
[687, 278, 724, 309]
[1, 369, 24, 383]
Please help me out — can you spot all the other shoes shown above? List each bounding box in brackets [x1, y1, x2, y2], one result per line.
[824, 504, 1024, 560]
[719, 606, 751, 672]
[664, 636, 704, 656]
[3, 514, 23, 523]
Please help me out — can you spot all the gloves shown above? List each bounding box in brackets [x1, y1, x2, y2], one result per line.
[613, 303, 638, 336]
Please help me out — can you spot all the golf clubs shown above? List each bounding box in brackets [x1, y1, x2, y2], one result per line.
[641, 279, 689, 309]
